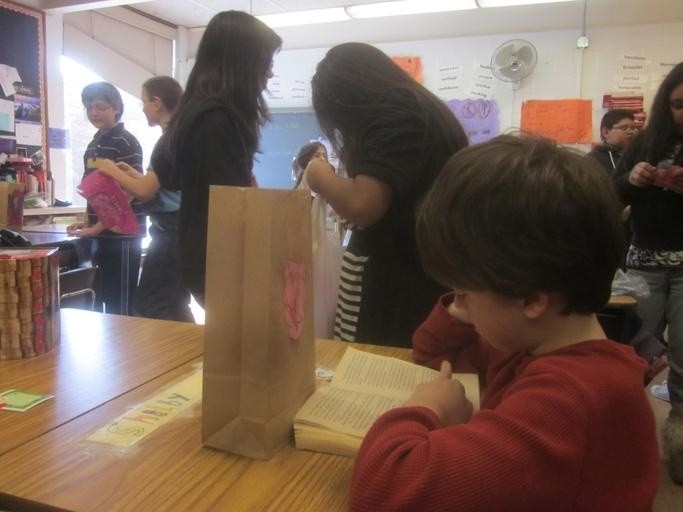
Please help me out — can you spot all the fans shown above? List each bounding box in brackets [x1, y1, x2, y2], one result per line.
[490, 39, 538, 90]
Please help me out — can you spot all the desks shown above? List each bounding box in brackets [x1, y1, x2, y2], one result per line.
[607, 294, 638, 343]
[22, 224, 87, 267]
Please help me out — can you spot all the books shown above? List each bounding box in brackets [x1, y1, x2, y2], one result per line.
[290, 346, 481, 458]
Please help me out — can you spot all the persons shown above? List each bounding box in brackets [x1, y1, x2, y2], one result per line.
[169, 8, 281, 326]
[289, 143, 330, 198]
[97, 73, 184, 319]
[303, 40, 470, 350]
[65, 81, 144, 316]
[581, 108, 636, 177]
[611, 61, 681, 486]
[343, 125, 659, 511]
[632, 110, 646, 131]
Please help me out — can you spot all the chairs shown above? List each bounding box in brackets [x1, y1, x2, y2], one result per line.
[59, 266, 104, 313]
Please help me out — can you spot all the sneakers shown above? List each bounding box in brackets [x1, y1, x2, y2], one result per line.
[651, 380, 670, 402]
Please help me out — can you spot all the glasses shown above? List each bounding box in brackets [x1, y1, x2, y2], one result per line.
[84, 104, 114, 113]
[608, 126, 635, 131]
